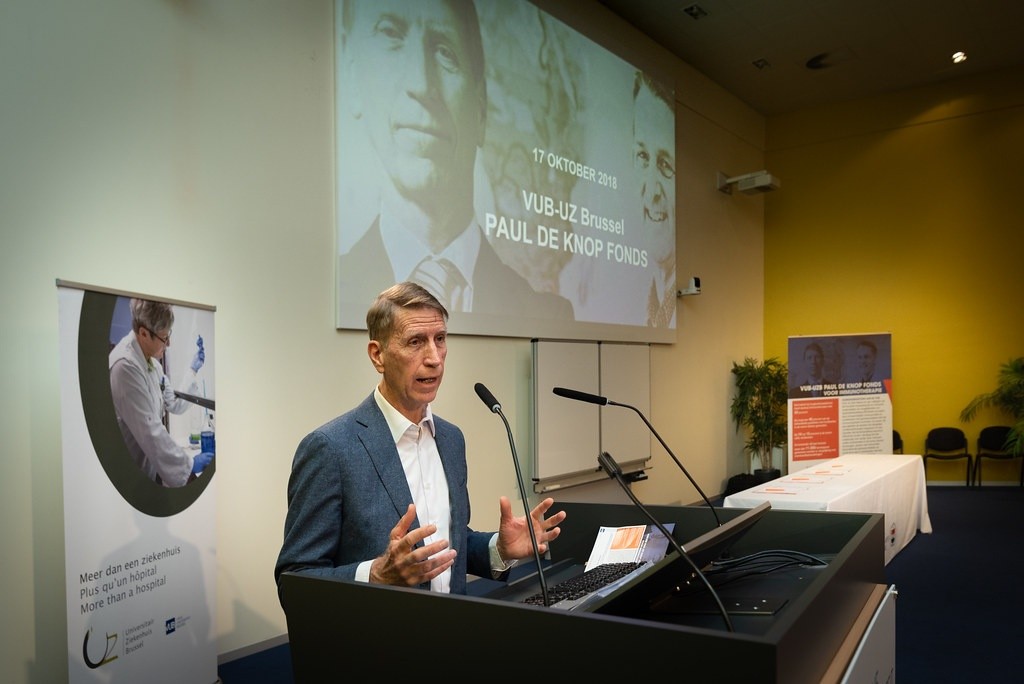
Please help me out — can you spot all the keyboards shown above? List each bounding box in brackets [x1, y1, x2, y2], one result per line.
[520, 560, 653, 607]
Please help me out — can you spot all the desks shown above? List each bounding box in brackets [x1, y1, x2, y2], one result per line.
[723, 453, 933, 567]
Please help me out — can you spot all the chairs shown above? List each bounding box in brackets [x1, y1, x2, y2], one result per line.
[893, 430, 903, 454]
[973, 426, 1024, 492]
[923, 427, 975, 487]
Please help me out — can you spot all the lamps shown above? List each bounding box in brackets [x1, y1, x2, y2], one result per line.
[714, 169, 781, 195]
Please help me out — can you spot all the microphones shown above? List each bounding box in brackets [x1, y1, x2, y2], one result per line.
[553, 387, 722, 528]
[474, 382, 548, 606]
[597, 451, 734, 632]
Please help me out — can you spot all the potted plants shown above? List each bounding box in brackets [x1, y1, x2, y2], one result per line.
[729, 355, 787, 485]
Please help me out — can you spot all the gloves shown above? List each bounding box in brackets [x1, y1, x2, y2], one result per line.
[192, 451, 214, 473]
[190, 351, 203, 371]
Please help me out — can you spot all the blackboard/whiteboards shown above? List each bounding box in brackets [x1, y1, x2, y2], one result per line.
[532, 337, 654, 494]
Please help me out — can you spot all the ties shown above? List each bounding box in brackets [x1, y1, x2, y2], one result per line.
[810, 381, 820, 397]
[860, 377, 866, 388]
[411, 257, 465, 315]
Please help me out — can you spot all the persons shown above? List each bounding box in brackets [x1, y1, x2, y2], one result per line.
[624, 74, 676, 329]
[789, 344, 834, 398]
[339, 0, 574, 320]
[275, 282, 566, 618]
[844, 341, 888, 393]
[108, 297, 213, 488]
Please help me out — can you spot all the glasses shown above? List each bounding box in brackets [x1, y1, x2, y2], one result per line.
[138, 324, 172, 345]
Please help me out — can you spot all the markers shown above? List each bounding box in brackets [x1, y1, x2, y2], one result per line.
[543, 484, 561, 491]
